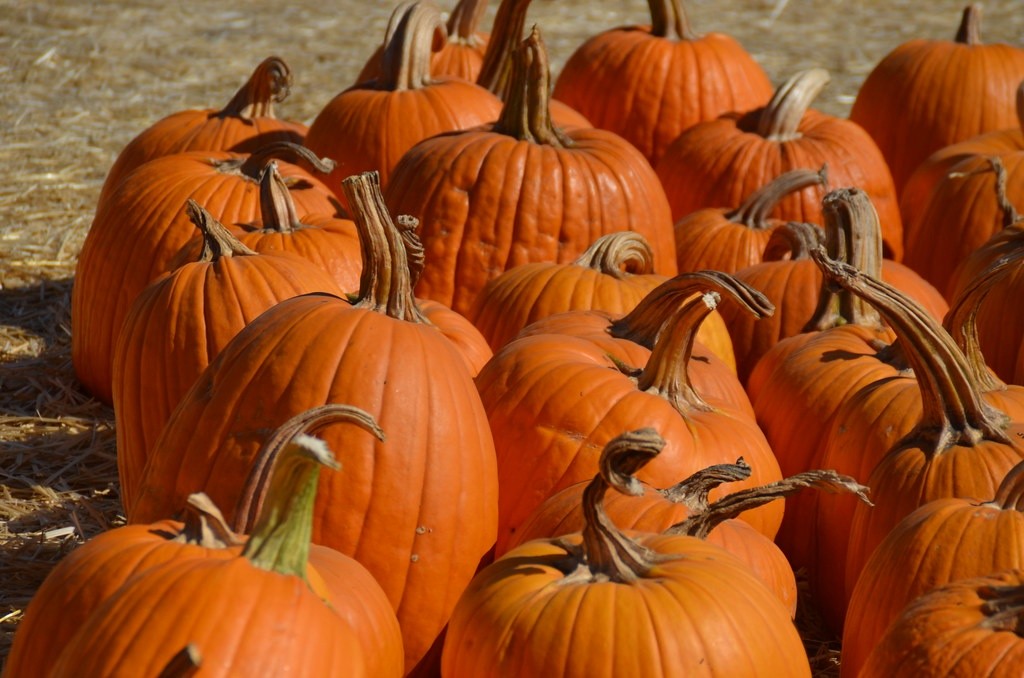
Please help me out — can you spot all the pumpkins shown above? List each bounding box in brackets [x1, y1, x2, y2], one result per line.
[0, 0, 1024, 678]
